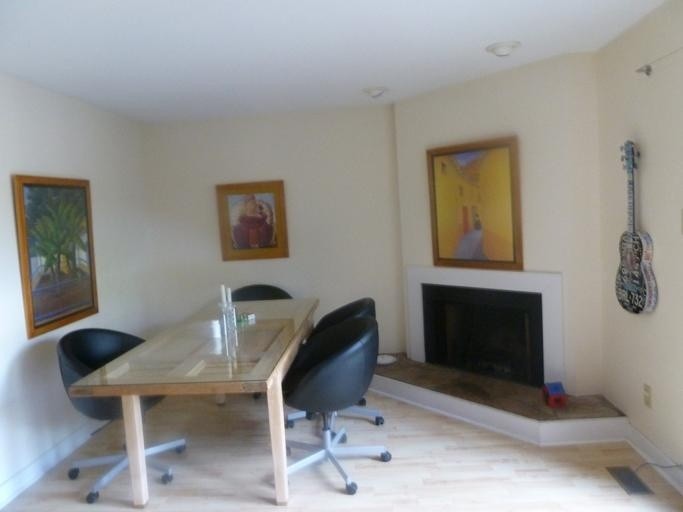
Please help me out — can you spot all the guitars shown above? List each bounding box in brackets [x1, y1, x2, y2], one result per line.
[613, 140, 660, 316]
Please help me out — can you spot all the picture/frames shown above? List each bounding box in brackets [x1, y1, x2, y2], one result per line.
[214, 180, 290, 262]
[424, 134, 524, 271]
[10, 174, 101, 342]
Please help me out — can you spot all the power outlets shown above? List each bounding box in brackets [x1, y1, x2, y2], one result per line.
[643, 384, 651, 408]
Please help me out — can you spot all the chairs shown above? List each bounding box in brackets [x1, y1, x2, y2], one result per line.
[57, 328, 188, 504]
[285, 297, 387, 442]
[230, 284, 293, 401]
[269, 317, 392, 494]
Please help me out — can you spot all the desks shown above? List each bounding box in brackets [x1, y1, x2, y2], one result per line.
[68, 296, 321, 507]
